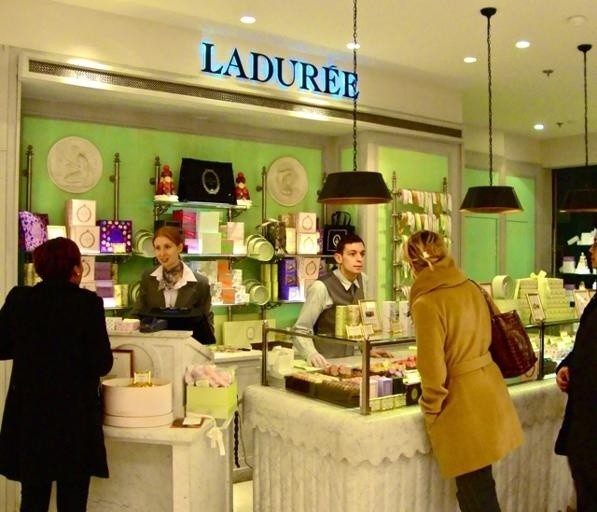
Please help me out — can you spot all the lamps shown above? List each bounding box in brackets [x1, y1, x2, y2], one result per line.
[557, 42, 597, 216]
[316, 1, 392, 206]
[458, 5, 526, 215]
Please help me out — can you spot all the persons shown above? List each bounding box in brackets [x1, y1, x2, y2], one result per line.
[406, 231, 525, 512]
[291, 233, 394, 369]
[0, 237, 114, 512]
[127, 226, 216, 345]
[554, 231, 597, 512]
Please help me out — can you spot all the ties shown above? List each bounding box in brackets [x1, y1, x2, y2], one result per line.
[348, 283, 359, 305]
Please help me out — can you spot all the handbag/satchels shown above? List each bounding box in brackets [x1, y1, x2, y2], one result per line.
[488, 309, 538, 378]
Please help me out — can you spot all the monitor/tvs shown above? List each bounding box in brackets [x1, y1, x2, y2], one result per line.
[135, 312, 216, 344]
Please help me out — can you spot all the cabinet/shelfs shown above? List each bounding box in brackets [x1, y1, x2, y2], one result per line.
[20, 143, 354, 356]
[51, 315, 265, 511]
[243, 373, 572, 510]
[385, 168, 455, 304]
